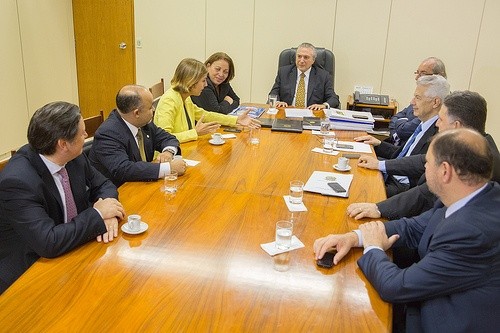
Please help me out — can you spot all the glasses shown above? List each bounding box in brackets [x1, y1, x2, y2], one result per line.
[414, 72, 438, 76]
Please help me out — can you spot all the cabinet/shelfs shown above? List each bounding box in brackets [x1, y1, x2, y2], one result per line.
[347, 94, 398, 141]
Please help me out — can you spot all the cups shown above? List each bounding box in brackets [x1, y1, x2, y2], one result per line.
[274, 252, 291, 272]
[250, 124, 261, 144]
[212, 133, 222, 143]
[338, 157, 348, 169]
[128, 214, 141, 232]
[163, 170, 177, 194]
[268, 94, 278, 111]
[321, 119, 331, 134]
[275, 220, 294, 251]
[289, 180, 304, 204]
[322, 134, 335, 153]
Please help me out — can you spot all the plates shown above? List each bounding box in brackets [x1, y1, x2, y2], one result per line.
[333, 164, 352, 172]
[209, 139, 225, 145]
[120, 221, 148, 235]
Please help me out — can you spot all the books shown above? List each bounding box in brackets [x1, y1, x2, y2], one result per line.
[271, 118, 303, 132]
[229, 107, 266, 118]
[303, 116, 321, 129]
[256, 118, 273, 128]
[329, 108, 375, 131]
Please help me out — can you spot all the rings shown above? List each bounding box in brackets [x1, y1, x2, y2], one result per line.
[359, 208, 362, 212]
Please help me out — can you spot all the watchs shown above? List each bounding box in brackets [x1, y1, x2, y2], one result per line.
[376, 205, 381, 216]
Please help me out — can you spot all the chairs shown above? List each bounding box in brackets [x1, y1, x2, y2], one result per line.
[0, 78, 165, 173]
[277, 48, 336, 94]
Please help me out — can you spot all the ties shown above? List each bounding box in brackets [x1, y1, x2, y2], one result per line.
[56, 167, 78, 223]
[137, 129, 146, 162]
[295, 73, 306, 107]
[384, 124, 422, 183]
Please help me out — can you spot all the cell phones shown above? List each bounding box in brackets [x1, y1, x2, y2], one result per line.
[317, 252, 336, 269]
[328, 182, 346, 192]
[223, 127, 241, 133]
[336, 143, 353, 150]
[342, 153, 362, 159]
[352, 115, 368, 119]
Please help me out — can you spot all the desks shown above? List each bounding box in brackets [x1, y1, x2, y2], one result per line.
[0, 102, 395, 333]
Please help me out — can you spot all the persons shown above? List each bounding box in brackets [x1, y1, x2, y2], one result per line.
[383, 57, 447, 145]
[0, 100, 126, 295]
[268, 42, 339, 110]
[312, 126, 500, 333]
[190, 52, 240, 126]
[353, 74, 450, 199]
[153, 58, 261, 161]
[88, 85, 187, 188]
[346, 90, 500, 219]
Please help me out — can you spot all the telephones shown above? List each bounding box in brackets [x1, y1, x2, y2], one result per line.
[353, 85, 373, 94]
[354, 90, 390, 106]
